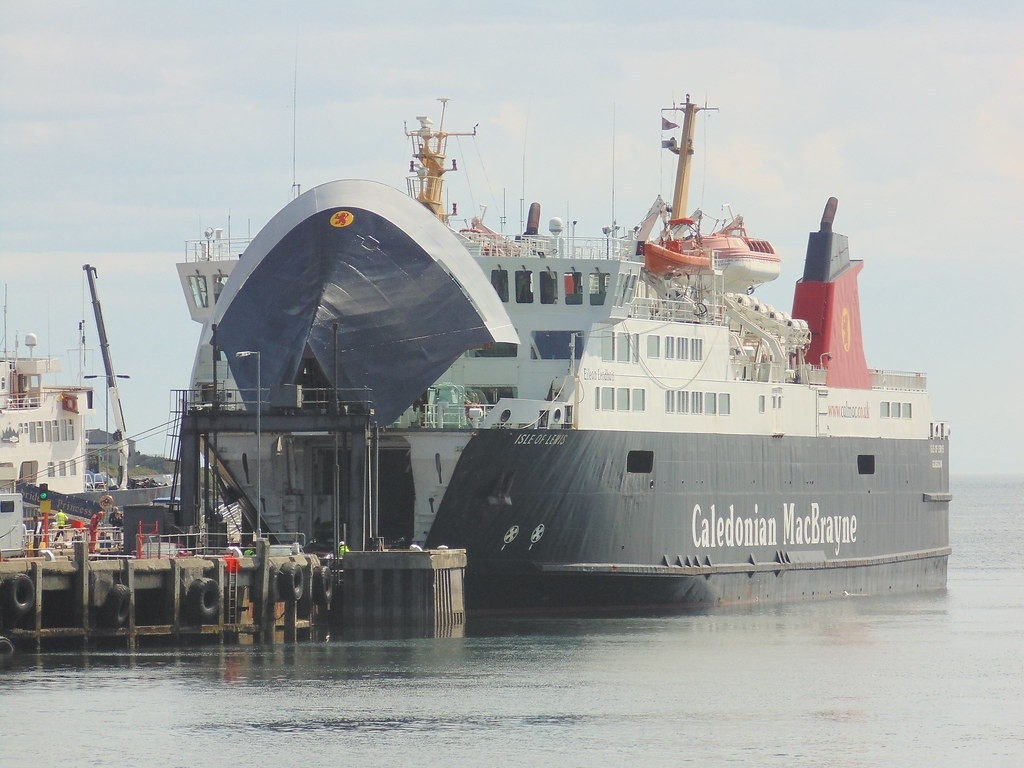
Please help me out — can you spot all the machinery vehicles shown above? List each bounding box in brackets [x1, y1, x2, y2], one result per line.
[78, 262, 131, 488]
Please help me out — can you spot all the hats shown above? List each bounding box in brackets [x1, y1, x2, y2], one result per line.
[58, 509, 62, 512]
[340, 541, 344, 545]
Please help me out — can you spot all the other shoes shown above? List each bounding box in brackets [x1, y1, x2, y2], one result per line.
[89, 552, 95, 553]
[113, 546, 116, 548]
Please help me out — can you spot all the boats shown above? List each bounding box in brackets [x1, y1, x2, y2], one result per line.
[171, 24, 953, 617]
[643, 207, 782, 295]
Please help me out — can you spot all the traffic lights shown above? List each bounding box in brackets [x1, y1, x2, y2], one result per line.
[37, 483, 48, 500]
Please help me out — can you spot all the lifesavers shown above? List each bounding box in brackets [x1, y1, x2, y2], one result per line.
[98, 580, 135, 631]
[309, 564, 339, 608]
[183, 573, 224, 624]
[99, 496, 114, 511]
[276, 559, 306, 606]
[3, 571, 41, 623]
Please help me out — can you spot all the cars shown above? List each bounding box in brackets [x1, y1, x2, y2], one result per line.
[132, 540, 189, 559]
[269, 543, 305, 556]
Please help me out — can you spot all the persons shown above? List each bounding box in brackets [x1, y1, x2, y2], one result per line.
[89, 511, 105, 554]
[53, 509, 68, 543]
[215, 277, 224, 304]
[33, 515, 43, 558]
[109, 505, 123, 548]
[338, 540, 349, 557]
[225, 539, 255, 559]
